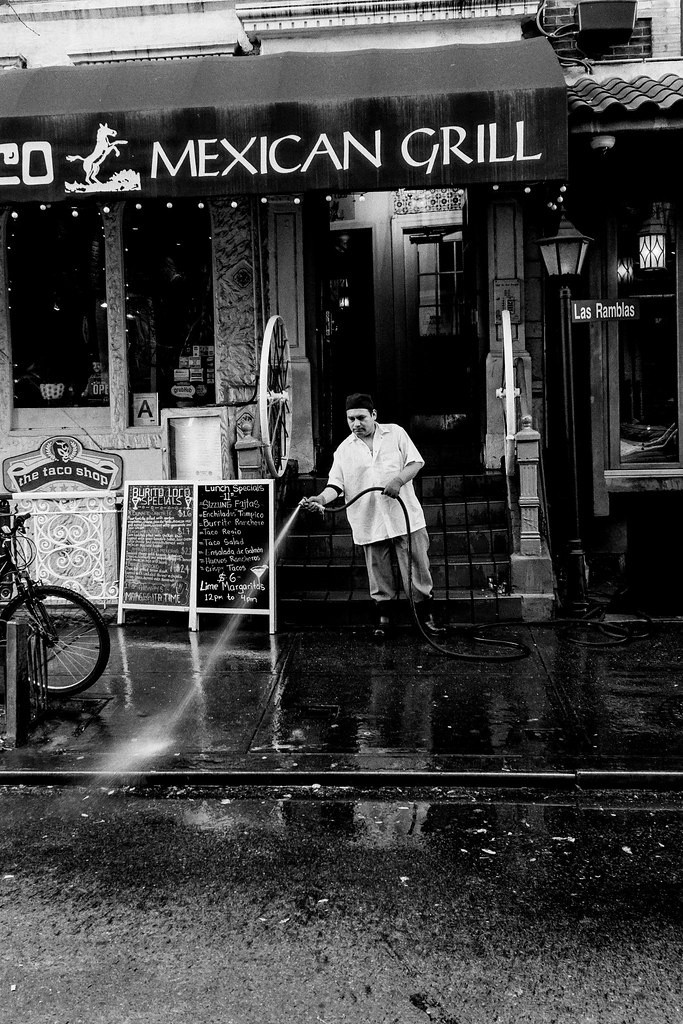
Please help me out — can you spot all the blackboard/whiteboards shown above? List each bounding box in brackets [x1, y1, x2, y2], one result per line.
[191, 479, 275, 615]
[116, 480, 199, 612]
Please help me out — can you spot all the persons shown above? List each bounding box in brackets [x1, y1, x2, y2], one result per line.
[302, 393, 447, 639]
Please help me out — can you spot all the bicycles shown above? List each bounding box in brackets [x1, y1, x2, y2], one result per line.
[0, 503, 110, 699]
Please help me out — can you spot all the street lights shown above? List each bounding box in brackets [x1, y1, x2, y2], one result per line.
[537, 209, 599, 622]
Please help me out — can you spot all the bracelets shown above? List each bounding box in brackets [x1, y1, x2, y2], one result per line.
[393, 477, 404, 487]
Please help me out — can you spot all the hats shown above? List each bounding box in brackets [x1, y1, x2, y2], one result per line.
[346, 393, 372, 410]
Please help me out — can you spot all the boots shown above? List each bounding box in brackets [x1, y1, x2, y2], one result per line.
[413, 601, 446, 636]
[374, 599, 395, 636]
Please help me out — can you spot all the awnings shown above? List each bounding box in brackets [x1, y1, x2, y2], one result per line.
[0, 37, 568, 201]
